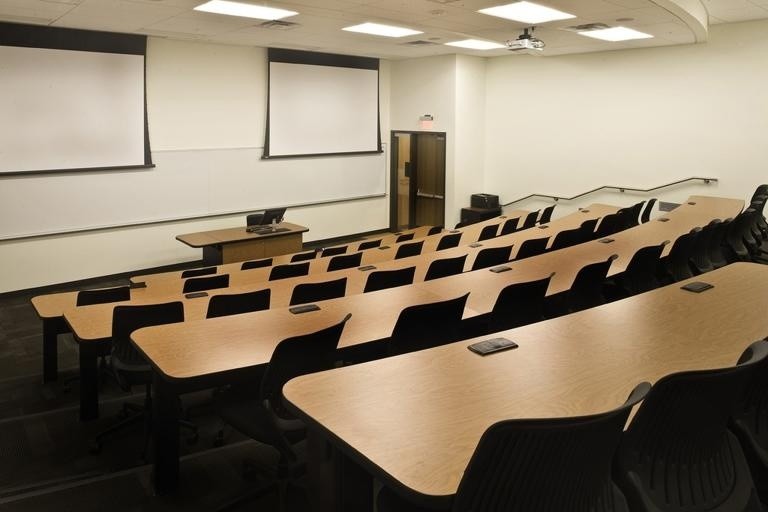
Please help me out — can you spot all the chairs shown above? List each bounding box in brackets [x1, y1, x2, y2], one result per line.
[71, 195, 768, 510]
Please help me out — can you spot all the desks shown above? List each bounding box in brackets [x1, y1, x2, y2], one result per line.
[30, 225, 450, 386]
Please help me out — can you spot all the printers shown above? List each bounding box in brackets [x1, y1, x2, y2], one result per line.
[471, 193, 499, 208]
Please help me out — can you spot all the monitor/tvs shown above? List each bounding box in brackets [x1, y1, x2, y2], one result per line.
[258, 207, 287, 232]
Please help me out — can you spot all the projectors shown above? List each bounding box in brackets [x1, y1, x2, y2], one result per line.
[505, 38, 545, 51]
